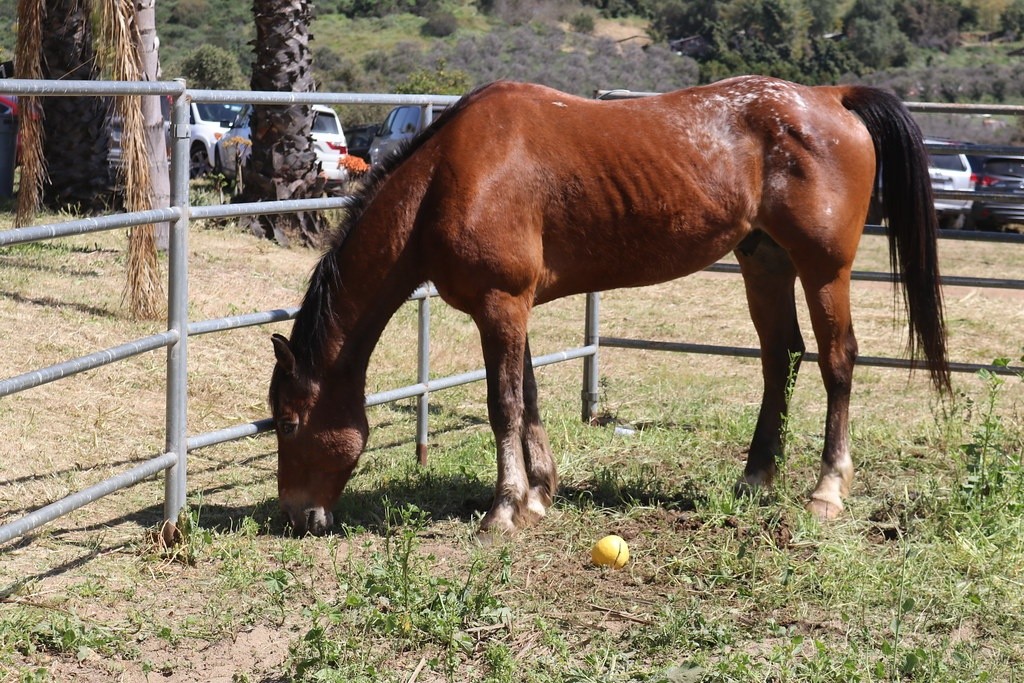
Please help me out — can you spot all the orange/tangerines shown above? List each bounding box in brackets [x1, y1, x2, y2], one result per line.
[592, 535, 629, 570]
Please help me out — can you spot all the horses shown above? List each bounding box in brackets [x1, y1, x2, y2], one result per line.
[269, 74, 964, 540]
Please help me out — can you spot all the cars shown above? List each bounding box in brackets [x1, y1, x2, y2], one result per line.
[0, 93, 46, 171]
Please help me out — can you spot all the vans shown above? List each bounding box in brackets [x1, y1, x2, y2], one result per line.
[104, 94, 172, 177]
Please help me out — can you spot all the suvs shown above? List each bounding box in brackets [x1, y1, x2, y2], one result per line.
[962, 143, 1024, 232]
[192, 101, 240, 180]
[870, 140, 979, 231]
[345, 103, 447, 170]
[215, 105, 348, 186]
